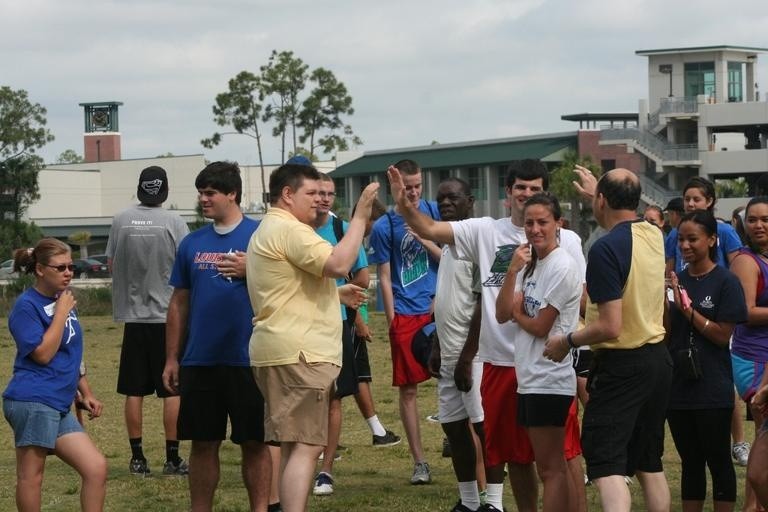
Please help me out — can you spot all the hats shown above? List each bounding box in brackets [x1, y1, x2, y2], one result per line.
[663, 197, 684, 211]
[137, 166, 167, 205]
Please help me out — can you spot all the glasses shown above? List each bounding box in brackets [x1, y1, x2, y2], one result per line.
[319, 191, 336, 198]
[45, 264, 77, 272]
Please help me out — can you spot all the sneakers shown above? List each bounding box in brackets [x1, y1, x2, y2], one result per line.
[625, 476, 632, 487]
[411, 463, 431, 484]
[129, 459, 150, 474]
[475, 503, 507, 512]
[164, 457, 189, 475]
[268, 502, 282, 512]
[584, 475, 592, 487]
[426, 414, 440, 422]
[373, 431, 401, 448]
[442, 438, 452, 457]
[450, 498, 474, 511]
[732, 441, 750, 466]
[313, 472, 334, 496]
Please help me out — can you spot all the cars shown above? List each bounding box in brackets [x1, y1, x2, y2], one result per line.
[0, 240, 114, 280]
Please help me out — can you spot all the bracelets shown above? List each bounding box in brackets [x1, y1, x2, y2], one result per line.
[700, 319, 710, 336]
[567, 333, 579, 348]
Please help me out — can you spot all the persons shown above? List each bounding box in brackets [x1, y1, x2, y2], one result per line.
[357, 158, 444, 485]
[109, 166, 192, 477]
[161, 161, 272, 510]
[1, 238, 110, 512]
[312, 171, 401, 496]
[385, 156, 766, 511]
[407, 176, 506, 510]
[245, 165, 379, 510]
